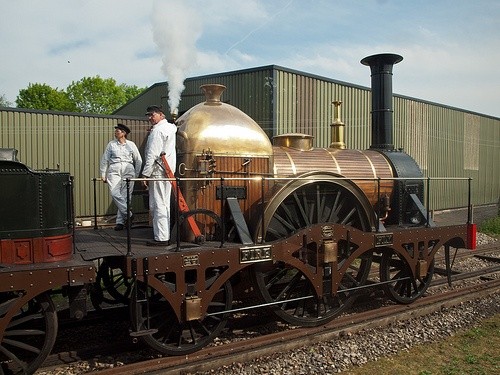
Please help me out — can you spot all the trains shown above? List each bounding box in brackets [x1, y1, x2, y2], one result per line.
[0, 53, 477, 375]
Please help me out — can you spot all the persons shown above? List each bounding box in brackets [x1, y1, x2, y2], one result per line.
[100, 123, 142, 232]
[142, 105, 177, 247]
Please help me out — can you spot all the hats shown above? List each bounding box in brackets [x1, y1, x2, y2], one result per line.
[114, 123, 131, 133]
[145, 105, 162, 116]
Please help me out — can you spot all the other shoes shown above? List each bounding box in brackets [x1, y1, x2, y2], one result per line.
[146, 238, 168, 246]
[114, 224, 124, 232]
